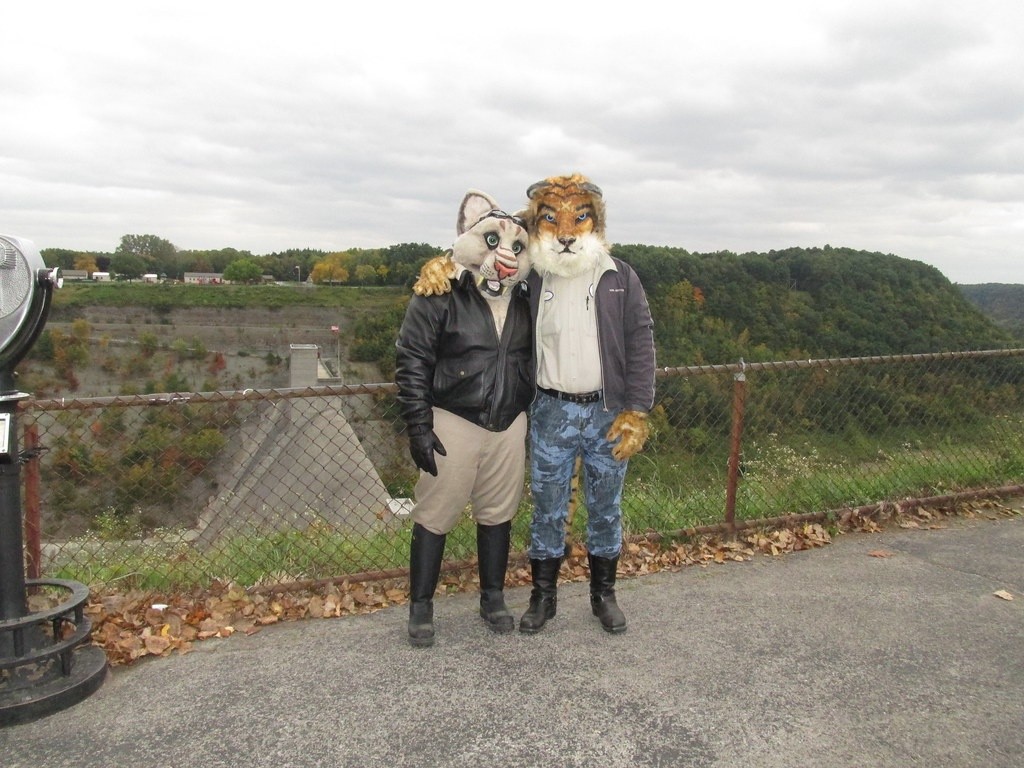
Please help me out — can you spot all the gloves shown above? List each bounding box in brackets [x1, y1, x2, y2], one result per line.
[407, 423, 448, 477]
[606, 409, 650, 463]
[412, 249, 457, 297]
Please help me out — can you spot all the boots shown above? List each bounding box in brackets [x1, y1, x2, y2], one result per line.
[476, 520, 515, 633]
[587, 551, 627, 633]
[407, 522, 446, 645]
[519, 557, 561, 634]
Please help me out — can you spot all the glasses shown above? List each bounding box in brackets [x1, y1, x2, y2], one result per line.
[468, 210, 528, 231]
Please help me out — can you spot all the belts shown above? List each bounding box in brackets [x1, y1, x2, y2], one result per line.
[536, 383, 603, 403]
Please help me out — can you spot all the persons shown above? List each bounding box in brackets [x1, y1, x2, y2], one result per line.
[413, 173, 656, 634]
[394, 189, 537, 645]
